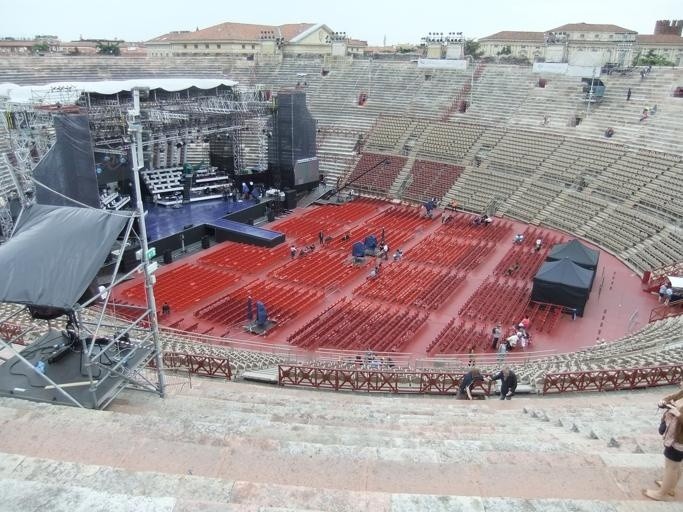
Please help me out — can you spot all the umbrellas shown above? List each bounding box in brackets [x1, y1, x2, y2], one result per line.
[248, 295, 253, 333]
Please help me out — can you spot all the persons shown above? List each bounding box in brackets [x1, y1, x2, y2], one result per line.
[162, 301, 170, 316]
[455, 314, 531, 401]
[536, 236, 542, 250]
[640, 369, 682, 501]
[602, 64, 657, 139]
[354, 349, 395, 369]
[657, 283, 668, 303]
[419, 197, 524, 244]
[506, 261, 519, 276]
[662, 287, 672, 306]
[289, 230, 402, 280]
[249, 180, 253, 200]
[241, 183, 249, 201]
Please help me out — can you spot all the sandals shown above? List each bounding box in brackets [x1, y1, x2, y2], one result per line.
[641, 488, 663, 501]
[654, 478, 676, 497]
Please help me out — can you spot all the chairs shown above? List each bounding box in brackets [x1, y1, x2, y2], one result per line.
[98, 116, 683, 391]
